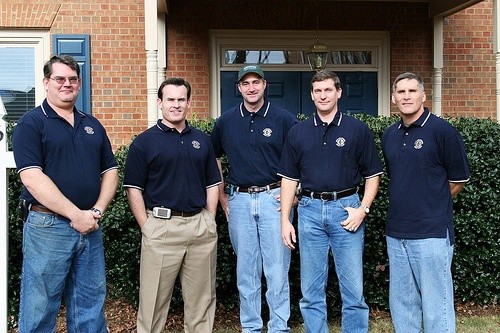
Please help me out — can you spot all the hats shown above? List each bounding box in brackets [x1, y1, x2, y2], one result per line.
[238, 63, 264, 82]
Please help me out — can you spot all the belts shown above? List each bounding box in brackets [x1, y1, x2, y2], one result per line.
[302, 187, 357, 201]
[150, 207, 206, 217]
[234, 181, 281, 194]
[31, 204, 63, 216]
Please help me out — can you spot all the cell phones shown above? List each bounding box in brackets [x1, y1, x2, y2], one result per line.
[152, 207, 171, 219]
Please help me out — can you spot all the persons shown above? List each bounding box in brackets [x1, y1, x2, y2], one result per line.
[13, 55, 119, 333]
[380, 72, 470, 333]
[211, 64, 301, 333]
[123, 78, 223, 333]
[277, 68, 384, 333]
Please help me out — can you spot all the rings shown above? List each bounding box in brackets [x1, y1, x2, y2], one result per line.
[352, 227, 355, 230]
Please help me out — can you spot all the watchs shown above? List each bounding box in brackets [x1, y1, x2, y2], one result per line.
[91, 208, 101, 215]
[363, 206, 369, 213]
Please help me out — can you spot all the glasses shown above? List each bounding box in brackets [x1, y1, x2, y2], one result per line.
[48, 77, 80, 84]
